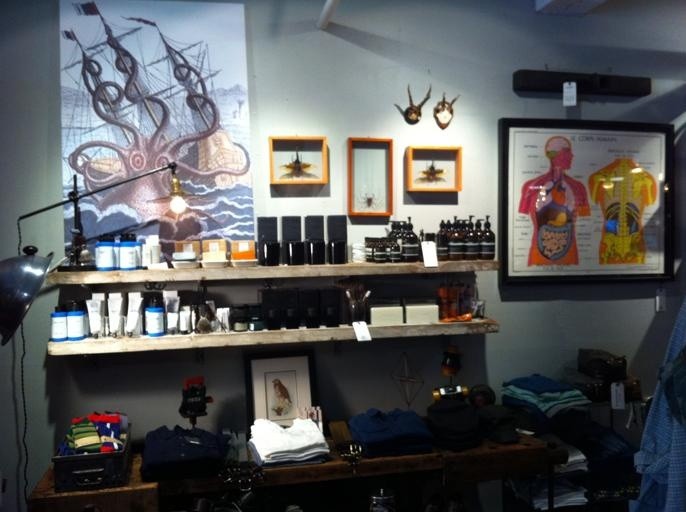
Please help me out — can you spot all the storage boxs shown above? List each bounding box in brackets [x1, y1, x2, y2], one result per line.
[52, 423, 133, 493]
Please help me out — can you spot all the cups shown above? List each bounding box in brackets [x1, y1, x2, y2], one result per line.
[349, 299, 368, 321]
[142, 245, 162, 271]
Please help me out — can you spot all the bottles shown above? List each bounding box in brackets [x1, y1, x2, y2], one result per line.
[50, 304, 88, 343]
[144, 306, 165, 337]
[437, 280, 475, 319]
[232, 316, 263, 332]
[93, 241, 144, 271]
[364, 236, 402, 263]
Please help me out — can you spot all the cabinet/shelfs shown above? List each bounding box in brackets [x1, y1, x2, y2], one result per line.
[47, 260, 501, 356]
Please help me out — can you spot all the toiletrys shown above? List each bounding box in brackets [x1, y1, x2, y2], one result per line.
[49, 215, 262, 343]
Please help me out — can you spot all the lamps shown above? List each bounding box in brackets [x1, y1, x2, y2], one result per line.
[0, 250, 53, 346]
[18, 162, 187, 270]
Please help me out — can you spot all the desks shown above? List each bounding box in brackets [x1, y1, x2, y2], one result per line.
[27, 435, 569, 512]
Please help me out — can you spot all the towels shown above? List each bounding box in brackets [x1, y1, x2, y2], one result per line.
[247, 418, 330, 466]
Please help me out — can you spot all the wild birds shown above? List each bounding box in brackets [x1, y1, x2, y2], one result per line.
[272, 378, 292, 412]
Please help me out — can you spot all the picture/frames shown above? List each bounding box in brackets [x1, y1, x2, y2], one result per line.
[347, 138, 393, 217]
[269, 136, 328, 185]
[243, 347, 319, 426]
[498, 118, 675, 287]
[406, 147, 462, 193]
